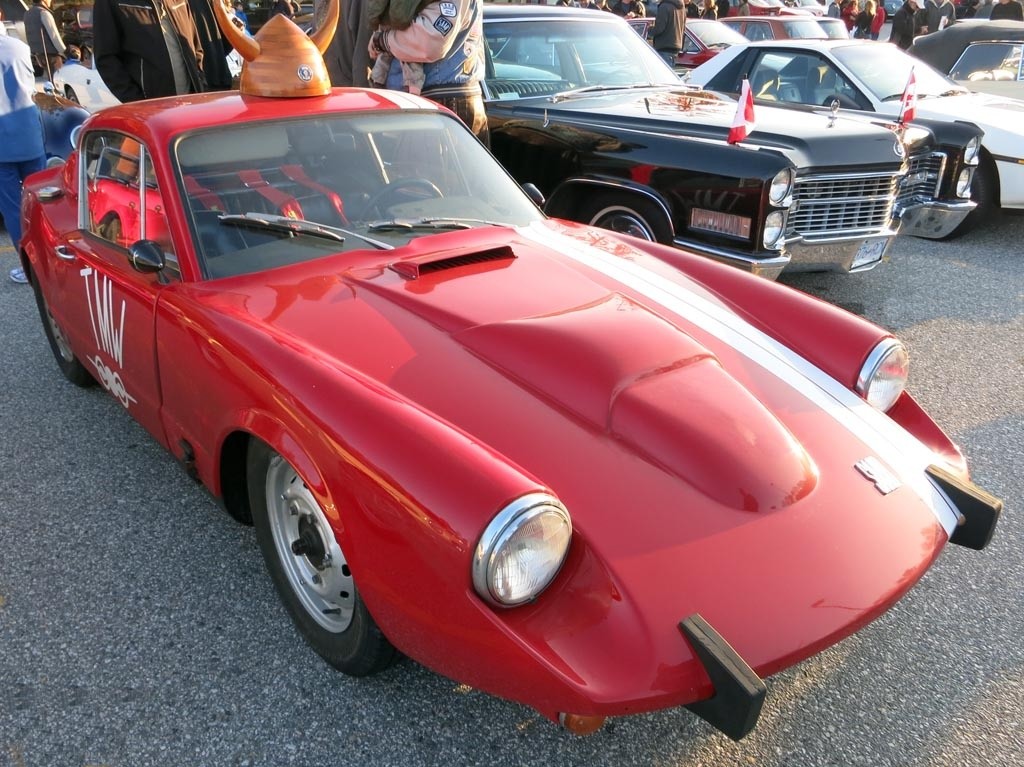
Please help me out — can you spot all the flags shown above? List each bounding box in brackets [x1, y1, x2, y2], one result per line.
[727, 79, 757, 145]
[900, 71, 917, 126]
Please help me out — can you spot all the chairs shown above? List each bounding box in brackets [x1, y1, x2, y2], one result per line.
[752, 70, 779, 101]
[807, 65, 836, 105]
[192, 211, 239, 259]
[277, 189, 380, 239]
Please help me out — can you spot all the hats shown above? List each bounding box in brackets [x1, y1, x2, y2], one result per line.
[910, 0, 926, 10]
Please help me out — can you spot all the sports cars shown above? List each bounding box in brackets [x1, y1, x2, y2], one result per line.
[17, 84, 1002, 742]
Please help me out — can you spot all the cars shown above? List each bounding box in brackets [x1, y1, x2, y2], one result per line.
[0, 0, 96, 52]
[679, 38, 1024, 214]
[905, 16, 1024, 104]
[622, 0, 850, 79]
[466, 4, 909, 285]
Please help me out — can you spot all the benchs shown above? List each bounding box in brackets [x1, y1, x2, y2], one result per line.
[190, 183, 346, 244]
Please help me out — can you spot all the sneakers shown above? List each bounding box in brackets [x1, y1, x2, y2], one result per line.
[8, 267, 30, 283]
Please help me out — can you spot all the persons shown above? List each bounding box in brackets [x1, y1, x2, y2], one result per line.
[0, 0, 487, 195]
[0, 23, 50, 284]
[556, 0, 1024, 66]
